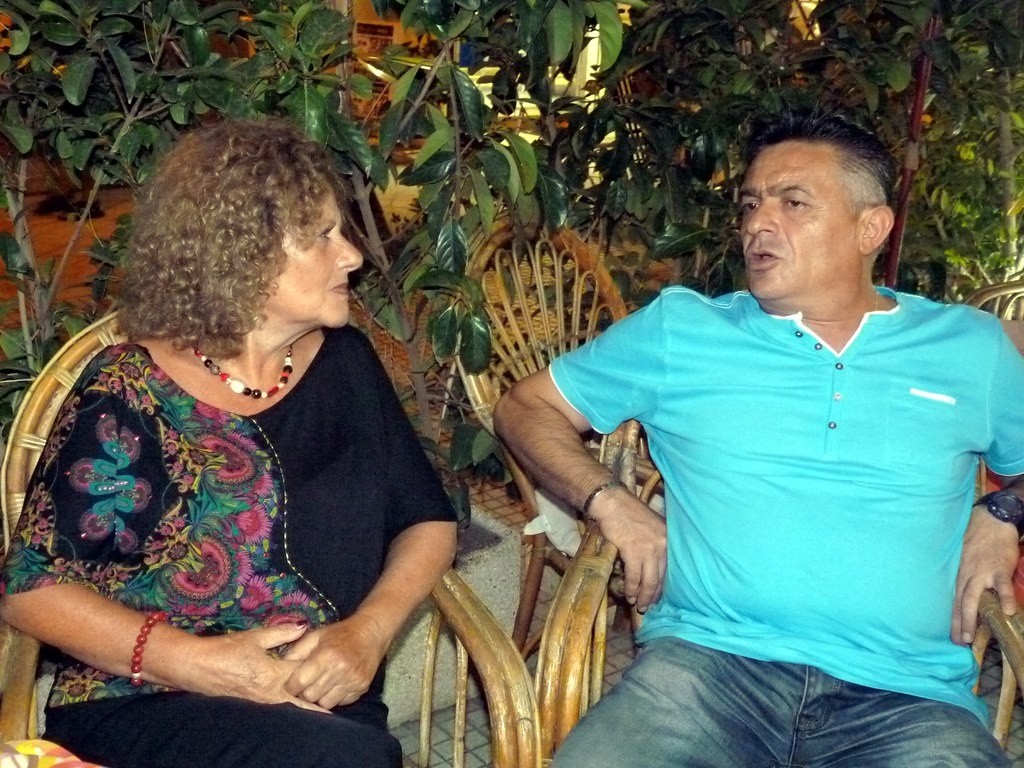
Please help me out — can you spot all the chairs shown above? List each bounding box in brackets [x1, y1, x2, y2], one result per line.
[0, 297, 543, 768]
[458, 215, 664, 663]
[534, 458, 1024, 768]
[962, 280, 1024, 699]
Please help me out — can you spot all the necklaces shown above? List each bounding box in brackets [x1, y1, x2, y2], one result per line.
[192, 344, 292, 398]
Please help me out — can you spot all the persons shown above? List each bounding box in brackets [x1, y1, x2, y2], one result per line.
[493, 110, 1024, 768]
[0, 116, 459, 768]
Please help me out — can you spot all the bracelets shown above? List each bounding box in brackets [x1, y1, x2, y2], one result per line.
[583, 481, 628, 522]
[131, 612, 168, 686]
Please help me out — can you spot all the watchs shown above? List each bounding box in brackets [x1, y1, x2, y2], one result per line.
[972, 491, 1024, 547]
